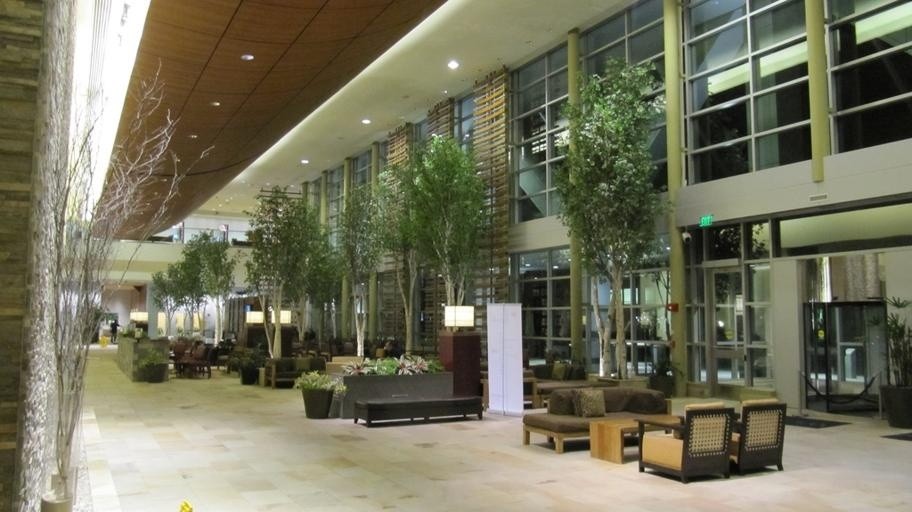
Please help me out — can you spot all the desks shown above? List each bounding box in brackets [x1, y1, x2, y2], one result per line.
[589, 413, 681, 465]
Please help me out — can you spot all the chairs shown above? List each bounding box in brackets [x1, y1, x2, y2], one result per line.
[633, 402, 741, 482]
[169, 336, 331, 389]
[730, 398, 788, 474]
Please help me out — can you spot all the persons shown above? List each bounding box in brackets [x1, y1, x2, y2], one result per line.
[110, 320, 120, 343]
[385, 341, 403, 358]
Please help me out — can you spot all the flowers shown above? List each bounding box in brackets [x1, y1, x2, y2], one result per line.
[342, 353, 446, 376]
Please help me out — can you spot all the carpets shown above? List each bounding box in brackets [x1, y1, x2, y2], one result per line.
[882, 432, 912, 442]
[782, 414, 852, 430]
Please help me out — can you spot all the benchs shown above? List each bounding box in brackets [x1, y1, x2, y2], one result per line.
[351, 395, 484, 427]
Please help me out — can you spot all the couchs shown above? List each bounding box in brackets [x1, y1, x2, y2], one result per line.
[524, 363, 610, 408]
[522, 386, 673, 454]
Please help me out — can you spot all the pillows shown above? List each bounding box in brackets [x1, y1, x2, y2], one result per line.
[572, 387, 592, 416]
[579, 388, 607, 417]
[564, 362, 572, 379]
[551, 362, 566, 380]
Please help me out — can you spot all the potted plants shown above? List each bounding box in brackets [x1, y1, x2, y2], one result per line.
[877, 311, 912, 430]
[295, 371, 337, 419]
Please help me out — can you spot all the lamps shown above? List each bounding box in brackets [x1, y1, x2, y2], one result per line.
[441, 303, 476, 331]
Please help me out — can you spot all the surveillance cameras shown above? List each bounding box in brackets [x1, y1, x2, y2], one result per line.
[681, 232, 693, 244]
